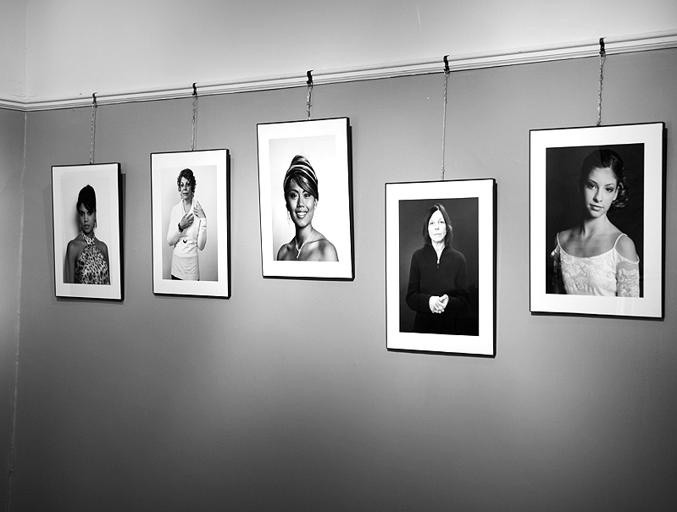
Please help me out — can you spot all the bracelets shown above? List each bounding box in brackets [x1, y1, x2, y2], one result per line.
[178, 224, 183, 232]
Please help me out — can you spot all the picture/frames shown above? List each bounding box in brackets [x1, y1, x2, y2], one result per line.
[530, 122, 668, 321]
[383, 178, 498, 358]
[50, 162, 125, 303]
[149, 148, 232, 299]
[256, 116, 355, 281]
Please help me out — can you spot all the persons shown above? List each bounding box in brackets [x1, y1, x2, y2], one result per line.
[550, 147, 640, 298]
[166, 168, 208, 281]
[276, 155, 338, 262]
[64, 185, 110, 286]
[406, 204, 473, 336]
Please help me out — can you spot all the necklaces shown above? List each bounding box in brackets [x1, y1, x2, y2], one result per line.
[294, 229, 314, 259]
[80, 231, 96, 245]
